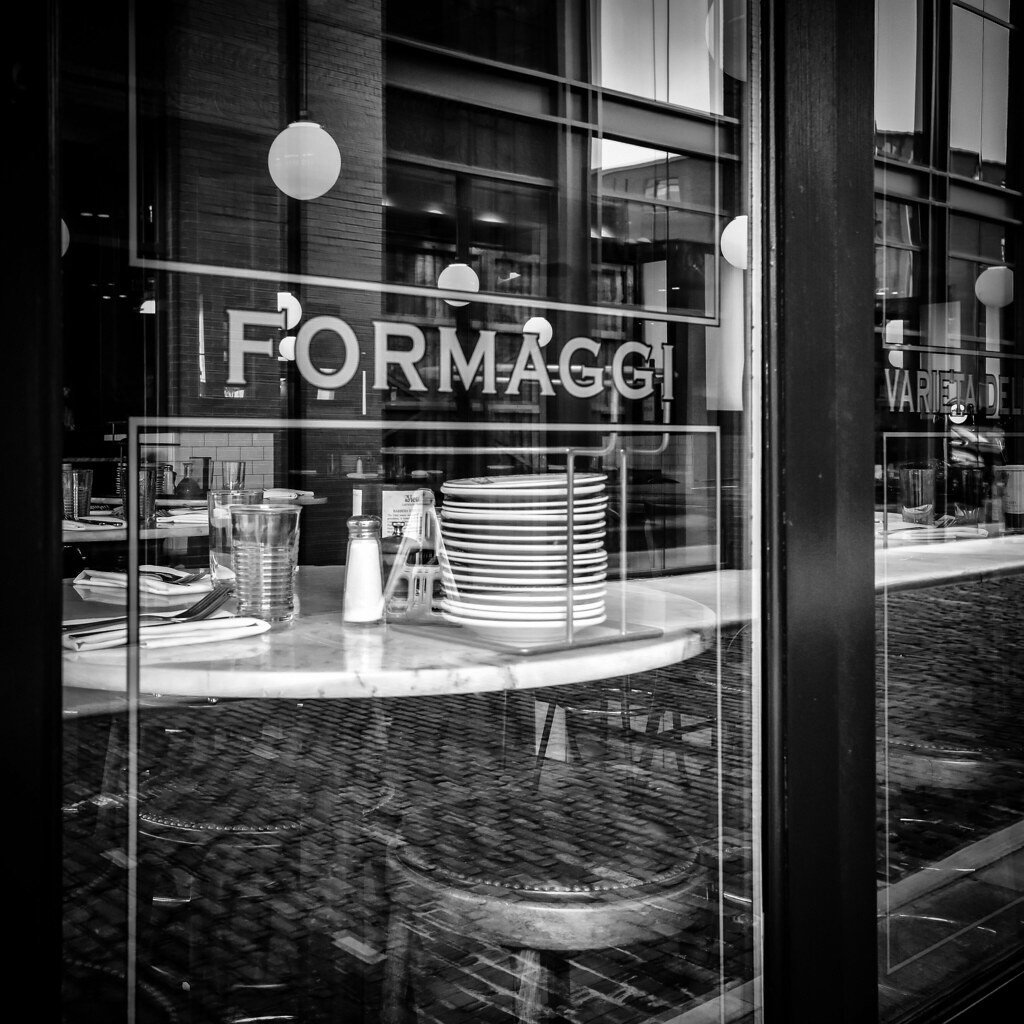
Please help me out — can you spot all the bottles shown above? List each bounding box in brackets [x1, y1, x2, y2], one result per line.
[339, 513, 385, 624]
[356, 456, 363, 474]
[176, 462, 201, 500]
[63, 464, 72, 470]
[164, 465, 174, 495]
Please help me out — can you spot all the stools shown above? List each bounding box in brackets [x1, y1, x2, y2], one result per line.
[84, 636, 1023, 1023]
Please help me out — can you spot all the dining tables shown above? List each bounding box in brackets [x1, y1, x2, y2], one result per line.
[61, 511, 210, 557]
[62, 565, 717, 698]
[91, 496, 328, 505]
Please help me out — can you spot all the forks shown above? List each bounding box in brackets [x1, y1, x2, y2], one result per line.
[137, 567, 209, 584]
[60, 582, 231, 635]
[878, 517, 953, 536]
[159, 510, 201, 517]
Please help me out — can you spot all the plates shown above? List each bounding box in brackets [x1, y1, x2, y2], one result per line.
[440, 469, 610, 647]
[115, 463, 165, 497]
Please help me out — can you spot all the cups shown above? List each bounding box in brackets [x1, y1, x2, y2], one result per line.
[120, 466, 158, 525]
[231, 504, 302, 614]
[381, 521, 419, 599]
[62, 470, 93, 520]
[206, 488, 263, 591]
[220, 460, 246, 491]
[188, 456, 214, 496]
[326, 454, 337, 476]
